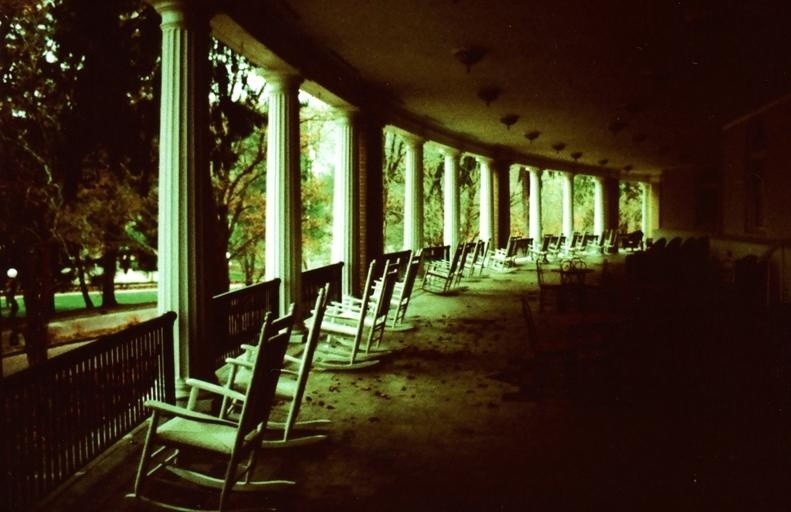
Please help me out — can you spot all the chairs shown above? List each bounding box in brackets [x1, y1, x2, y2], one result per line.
[425, 238, 491, 294]
[520, 295, 575, 402]
[533, 260, 567, 313]
[327, 248, 424, 354]
[215, 282, 335, 448]
[488, 228, 619, 273]
[124, 301, 297, 512]
[301, 258, 379, 369]
